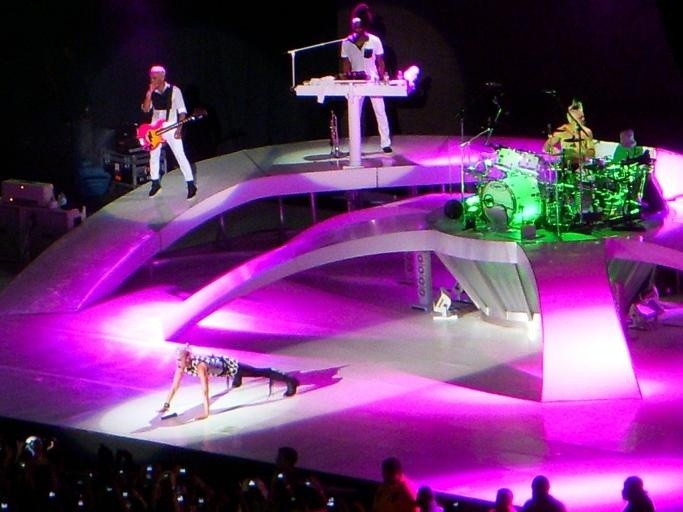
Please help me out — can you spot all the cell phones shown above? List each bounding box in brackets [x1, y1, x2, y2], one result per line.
[0, 460, 335, 512]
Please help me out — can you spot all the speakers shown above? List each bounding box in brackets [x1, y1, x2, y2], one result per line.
[0, 203, 29, 263]
[29, 207, 80, 260]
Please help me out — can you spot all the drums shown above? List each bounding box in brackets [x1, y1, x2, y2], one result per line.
[479, 175, 542, 229]
[493, 148, 539, 176]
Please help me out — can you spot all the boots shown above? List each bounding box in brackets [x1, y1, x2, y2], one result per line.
[187, 181, 196, 198]
[149, 180, 161, 196]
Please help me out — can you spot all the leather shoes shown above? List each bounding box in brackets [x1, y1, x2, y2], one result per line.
[285, 379, 298, 396]
[232, 376, 242, 386]
[383, 147, 392, 152]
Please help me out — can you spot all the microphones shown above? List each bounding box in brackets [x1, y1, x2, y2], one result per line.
[485, 82, 503, 87]
[348, 33, 360, 43]
[160, 412, 178, 420]
[545, 90, 559, 94]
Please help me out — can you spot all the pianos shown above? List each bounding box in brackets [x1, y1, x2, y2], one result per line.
[295, 76, 416, 97]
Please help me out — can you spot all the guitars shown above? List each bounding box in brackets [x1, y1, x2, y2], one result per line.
[136, 109, 207, 151]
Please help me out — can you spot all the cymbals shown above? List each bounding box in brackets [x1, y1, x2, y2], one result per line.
[553, 151, 577, 155]
[540, 127, 566, 131]
[563, 138, 584, 142]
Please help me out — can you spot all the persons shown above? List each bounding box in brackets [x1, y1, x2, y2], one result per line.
[141, 66, 197, 201]
[612, 128, 644, 166]
[544, 99, 595, 171]
[340, 4, 393, 155]
[491, 475, 565, 512]
[373, 457, 439, 512]
[155, 346, 300, 419]
[622, 476, 655, 512]
[1, 435, 330, 512]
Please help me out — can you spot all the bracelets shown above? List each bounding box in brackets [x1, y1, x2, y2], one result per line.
[164, 403, 169, 408]
